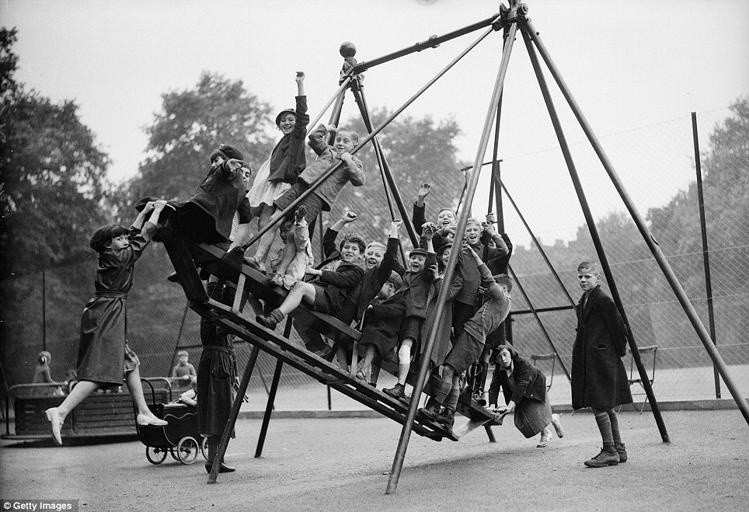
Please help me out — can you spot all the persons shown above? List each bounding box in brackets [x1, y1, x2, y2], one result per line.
[167, 70, 565, 448]
[29, 351, 62, 396]
[569, 260, 635, 470]
[40, 199, 168, 448]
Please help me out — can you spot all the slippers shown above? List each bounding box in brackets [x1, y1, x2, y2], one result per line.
[243, 256, 266, 275]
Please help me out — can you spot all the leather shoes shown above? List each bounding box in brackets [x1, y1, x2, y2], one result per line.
[256, 315, 277, 331]
[136, 414, 169, 426]
[44, 408, 63, 445]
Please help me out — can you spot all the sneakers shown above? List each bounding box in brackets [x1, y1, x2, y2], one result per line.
[382, 383, 404, 398]
[315, 344, 331, 357]
[356, 370, 366, 379]
[553, 413, 564, 438]
[271, 275, 284, 286]
[537, 432, 551, 447]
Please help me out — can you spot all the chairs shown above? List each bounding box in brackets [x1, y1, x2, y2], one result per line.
[531, 353, 557, 391]
[618, 345, 658, 416]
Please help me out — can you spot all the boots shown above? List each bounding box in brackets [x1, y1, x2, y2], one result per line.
[437, 407, 455, 431]
[585, 444, 626, 467]
[419, 397, 440, 419]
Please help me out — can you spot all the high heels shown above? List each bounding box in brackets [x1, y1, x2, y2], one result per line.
[204, 460, 236, 474]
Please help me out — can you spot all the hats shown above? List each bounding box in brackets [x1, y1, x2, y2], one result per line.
[409, 248, 429, 256]
[218, 144, 243, 165]
[276, 109, 297, 124]
[437, 242, 452, 260]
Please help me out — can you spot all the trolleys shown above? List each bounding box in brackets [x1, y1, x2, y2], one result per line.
[124, 377, 235, 464]
